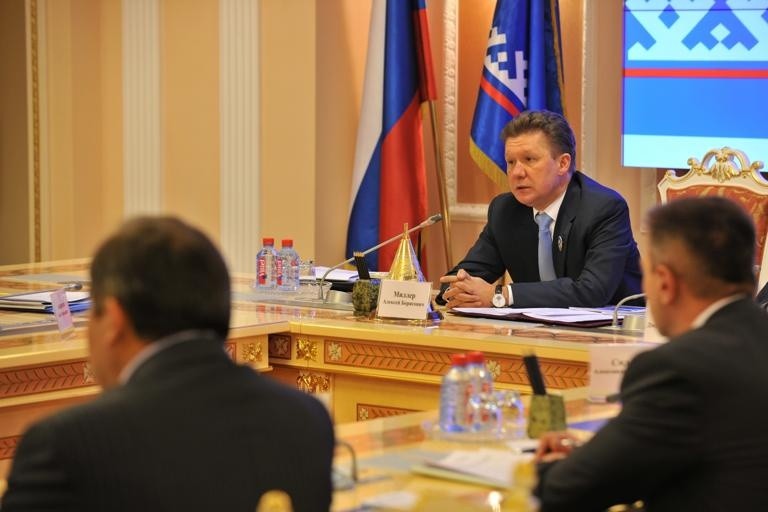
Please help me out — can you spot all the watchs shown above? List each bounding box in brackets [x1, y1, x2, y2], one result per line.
[491, 283, 506, 308]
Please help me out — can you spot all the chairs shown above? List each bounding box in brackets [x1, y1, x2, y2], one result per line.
[656, 146, 768, 300]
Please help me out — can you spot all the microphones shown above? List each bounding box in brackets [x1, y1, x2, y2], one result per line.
[318, 211, 446, 300]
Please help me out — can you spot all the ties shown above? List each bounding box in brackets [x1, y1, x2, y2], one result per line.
[534, 212, 557, 282]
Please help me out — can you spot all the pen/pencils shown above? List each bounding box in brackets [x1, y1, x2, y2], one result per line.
[64, 284, 83, 291]
[521, 446, 573, 453]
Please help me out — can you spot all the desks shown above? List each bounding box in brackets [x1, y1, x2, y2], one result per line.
[0, 276, 669, 512]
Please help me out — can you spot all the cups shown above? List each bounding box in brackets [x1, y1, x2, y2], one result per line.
[464, 390, 523, 439]
[299, 257, 317, 276]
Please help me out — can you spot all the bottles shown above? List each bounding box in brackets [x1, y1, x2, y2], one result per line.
[256, 238, 299, 291]
[440, 350, 492, 433]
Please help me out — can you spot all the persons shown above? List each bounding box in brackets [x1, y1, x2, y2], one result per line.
[434, 108, 646, 313]
[0, 215, 333, 511]
[527, 194, 768, 511]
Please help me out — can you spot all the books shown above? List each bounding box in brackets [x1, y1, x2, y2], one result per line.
[0, 288, 90, 310]
[446, 305, 624, 329]
[411, 447, 535, 489]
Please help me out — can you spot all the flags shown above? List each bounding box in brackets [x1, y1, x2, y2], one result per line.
[467, 0, 565, 194]
[344, 0, 438, 283]
